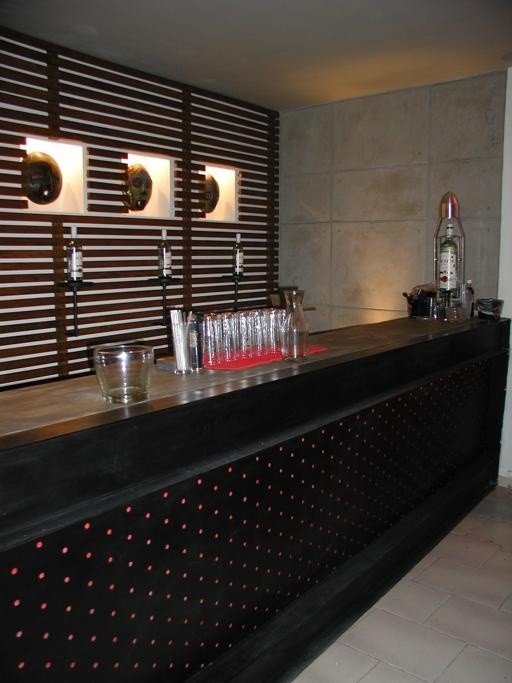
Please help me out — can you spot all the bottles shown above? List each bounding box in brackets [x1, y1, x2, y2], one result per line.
[281, 289, 310, 360]
[466, 280, 475, 318]
[159, 229, 172, 277]
[439, 218, 460, 297]
[66, 226, 83, 281]
[233, 233, 244, 274]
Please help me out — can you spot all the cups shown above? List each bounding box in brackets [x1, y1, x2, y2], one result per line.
[93, 345, 155, 404]
[203, 307, 287, 368]
